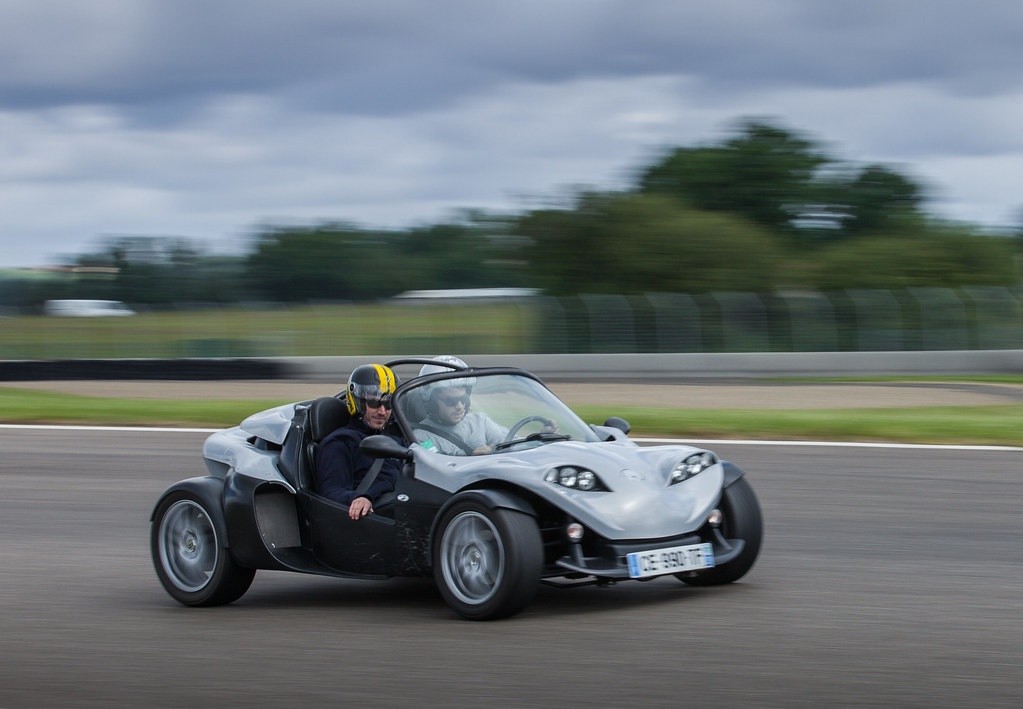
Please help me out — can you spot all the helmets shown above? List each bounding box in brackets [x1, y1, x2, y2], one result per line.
[345, 364, 400, 416]
[418, 355, 476, 401]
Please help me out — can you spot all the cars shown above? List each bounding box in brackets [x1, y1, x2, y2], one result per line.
[149, 358, 765, 621]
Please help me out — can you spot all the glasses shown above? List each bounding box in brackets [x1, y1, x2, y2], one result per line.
[437, 394, 468, 407]
[367, 399, 393, 410]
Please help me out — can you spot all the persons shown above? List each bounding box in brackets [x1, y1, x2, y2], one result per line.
[314, 364, 447, 520]
[410, 355, 560, 455]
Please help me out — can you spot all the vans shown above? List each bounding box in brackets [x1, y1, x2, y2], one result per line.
[50, 299, 135, 318]
